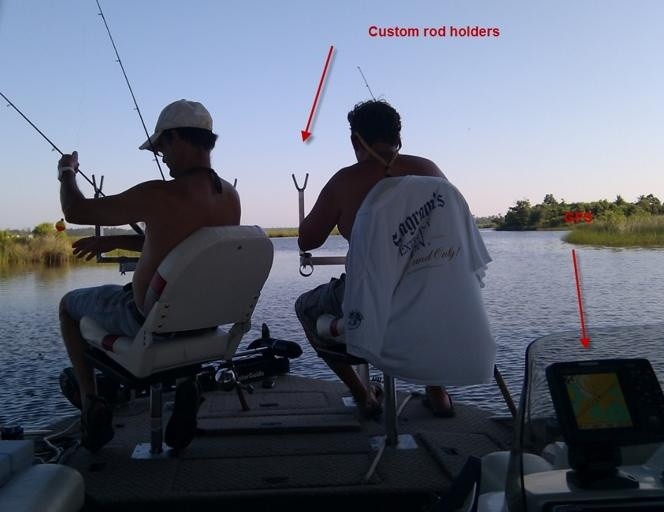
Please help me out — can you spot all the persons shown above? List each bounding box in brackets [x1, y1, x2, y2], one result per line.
[298, 101, 455, 418]
[58, 100, 241, 448]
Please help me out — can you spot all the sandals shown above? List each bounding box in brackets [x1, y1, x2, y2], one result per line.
[165, 382, 206, 449]
[81, 395, 114, 451]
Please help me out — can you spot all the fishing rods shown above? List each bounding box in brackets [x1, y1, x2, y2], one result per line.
[0, 91, 144, 235]
[356, 65, 377, 103]
[95, 0, 165, 180]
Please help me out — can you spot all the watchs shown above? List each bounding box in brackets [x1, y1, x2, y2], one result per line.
[58, 167, 76, 182]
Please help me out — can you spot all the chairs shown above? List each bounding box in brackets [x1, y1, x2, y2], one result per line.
[315, 175, 469, 451]
[80, 226, 274, 460]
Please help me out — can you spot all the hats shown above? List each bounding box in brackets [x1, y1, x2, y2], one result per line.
[139, 99, 212, 152]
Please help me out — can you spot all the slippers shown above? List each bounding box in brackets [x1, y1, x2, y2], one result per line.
[367, 380, 383, 418]
[423, 390, 456, 418]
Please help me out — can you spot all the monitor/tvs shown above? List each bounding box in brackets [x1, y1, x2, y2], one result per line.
[546, 358, 663, 445]
